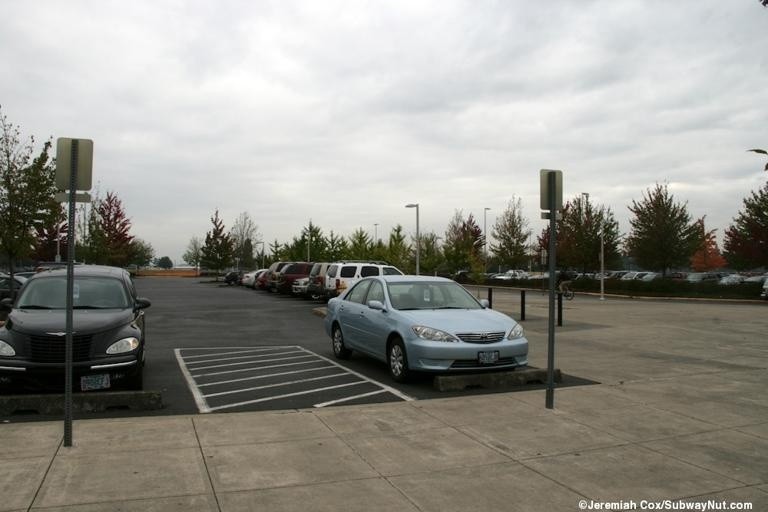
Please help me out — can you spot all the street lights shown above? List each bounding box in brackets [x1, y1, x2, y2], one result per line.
[483, 207, 492, 273]
[529, 228, 534, 271]
[306, 232, 311, 261]
[600, 220, 612, 297]
[374, 223, 380, 243]
[405, 204, 420, 275]
[256, 242, 264, 269]
[581, 193, 589, 218]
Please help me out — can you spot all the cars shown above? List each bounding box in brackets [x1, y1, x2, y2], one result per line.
[326, 274, 528, 384]
[0, 265, 152, 392]
[224, 268, 268, 289]
[452, 269, 767, 301]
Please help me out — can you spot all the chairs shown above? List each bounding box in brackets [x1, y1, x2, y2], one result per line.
[398, 294, 417, 307]
[100, 285, 124, 305]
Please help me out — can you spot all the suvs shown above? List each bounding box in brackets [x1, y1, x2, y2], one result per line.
[266, 260, 415, 302]
